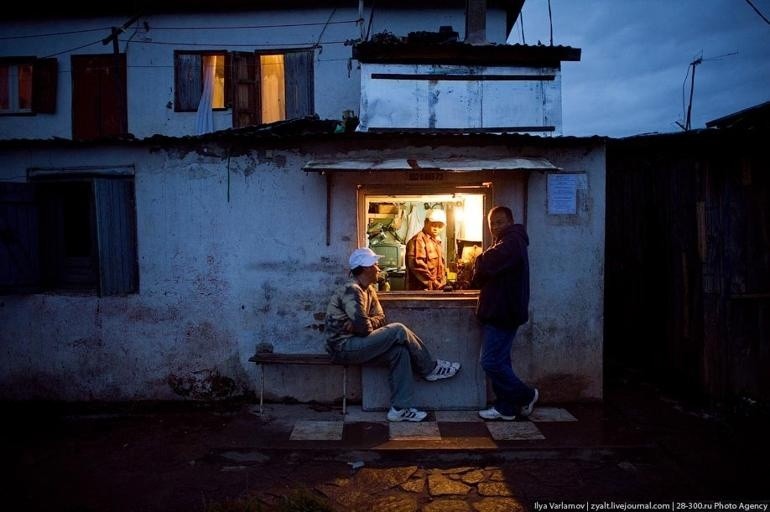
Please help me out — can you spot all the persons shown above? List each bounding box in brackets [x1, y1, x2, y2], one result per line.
[322, 247, 463, 423]
[404, 206, 449, 290]
[471, 204, 541, 421]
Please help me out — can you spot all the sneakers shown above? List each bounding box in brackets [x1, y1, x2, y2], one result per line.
[479, 407, 517, 421]
[425, 359, 462, 382]
[521, 389, 540, 419]
[387, 406, 428, 423]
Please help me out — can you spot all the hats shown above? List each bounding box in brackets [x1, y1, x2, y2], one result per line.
[426, 209, 447, 223]
[349, 248, 386, 270]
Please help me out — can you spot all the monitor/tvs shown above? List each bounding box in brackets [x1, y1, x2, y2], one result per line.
[370, 244, 400, 268]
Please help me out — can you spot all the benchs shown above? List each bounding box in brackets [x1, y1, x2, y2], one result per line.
[248, 352, 348, 415]
